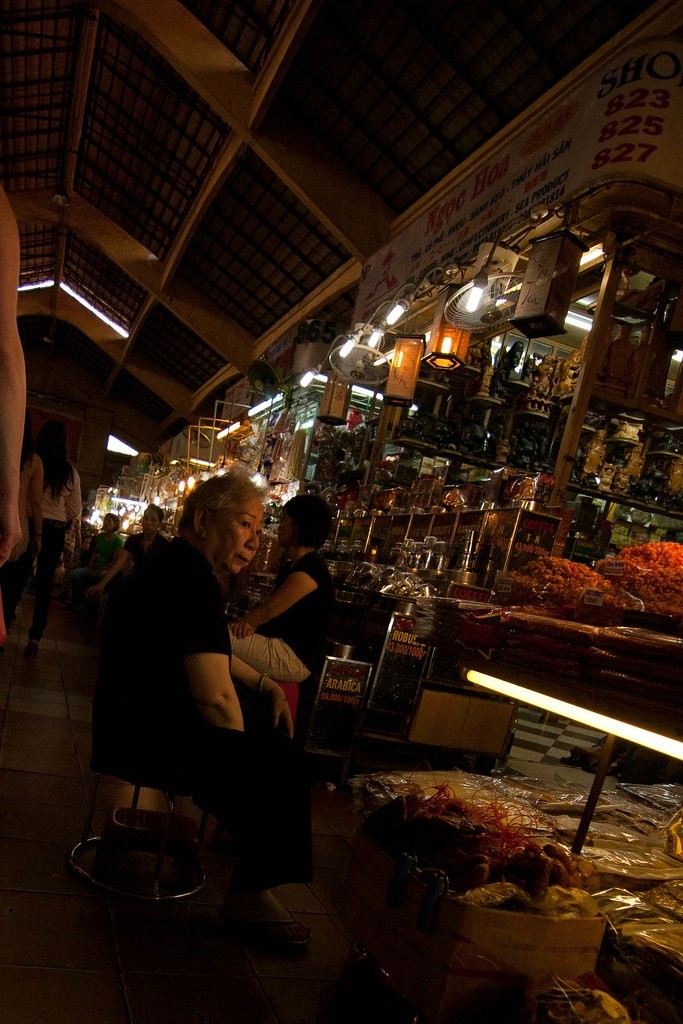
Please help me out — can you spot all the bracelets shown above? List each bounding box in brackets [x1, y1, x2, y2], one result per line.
[259, 672, 270, 695]
[35, 532, 42, 537]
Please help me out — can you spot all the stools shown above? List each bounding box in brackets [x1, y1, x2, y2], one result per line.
[67, 758, 209, 902]
[274, 679, 299, 729]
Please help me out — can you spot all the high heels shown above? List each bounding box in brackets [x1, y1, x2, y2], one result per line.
[6, 629, 11, 635]
[24, 641, 39, 658]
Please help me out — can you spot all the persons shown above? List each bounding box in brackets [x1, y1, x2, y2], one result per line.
[89, 473, 313, 948]
[1, 181, 24, 576]
[223, 495, 334, 683]
[10, 420, 82, 656]
[0, 407, 44, 654]
[57, 504, 168, 643]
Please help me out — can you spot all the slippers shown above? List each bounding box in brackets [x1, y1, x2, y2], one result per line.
[218, 906, 313, 944]
[210, 837, 246, 852]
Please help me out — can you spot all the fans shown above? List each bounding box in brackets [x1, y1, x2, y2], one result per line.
[248, 359, 280, 403]
[329, 322, 390, 386]
[442, 239, 524, 332]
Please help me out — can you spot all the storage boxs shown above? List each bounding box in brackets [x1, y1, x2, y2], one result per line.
[561, 519, 683, 568]
[342, 826, 607, 1024]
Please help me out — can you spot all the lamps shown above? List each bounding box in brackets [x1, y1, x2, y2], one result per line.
[297, 265, 458, 388]
[213, 390, 284, 441]
[456, 660, 682, 765]
